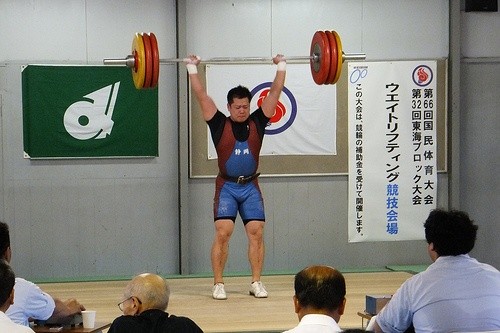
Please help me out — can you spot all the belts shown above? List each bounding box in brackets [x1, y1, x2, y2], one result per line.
[219, 172, 260, 185]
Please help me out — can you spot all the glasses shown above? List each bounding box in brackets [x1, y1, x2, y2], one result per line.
[118, 296, 142, 312]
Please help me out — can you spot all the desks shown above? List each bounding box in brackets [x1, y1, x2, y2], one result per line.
[29, 317, 112, 333]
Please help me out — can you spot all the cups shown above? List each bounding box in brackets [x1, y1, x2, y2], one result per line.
[81, 310, 96, 329]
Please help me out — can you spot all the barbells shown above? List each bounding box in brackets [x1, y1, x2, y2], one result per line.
[104, 30, 368, 90]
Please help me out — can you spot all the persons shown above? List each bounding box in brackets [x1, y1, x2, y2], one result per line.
[184, 53, 288, 300]
[0, 221, 85, 326]
[0, 259, 37, 333]
[107, 273, 205, 333]
[373, 208, 500, 332]
[281, 265, 348, 333]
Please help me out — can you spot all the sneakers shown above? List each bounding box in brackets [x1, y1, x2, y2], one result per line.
[249, 281, 268, 298]
[213, 283, 227, 300]
